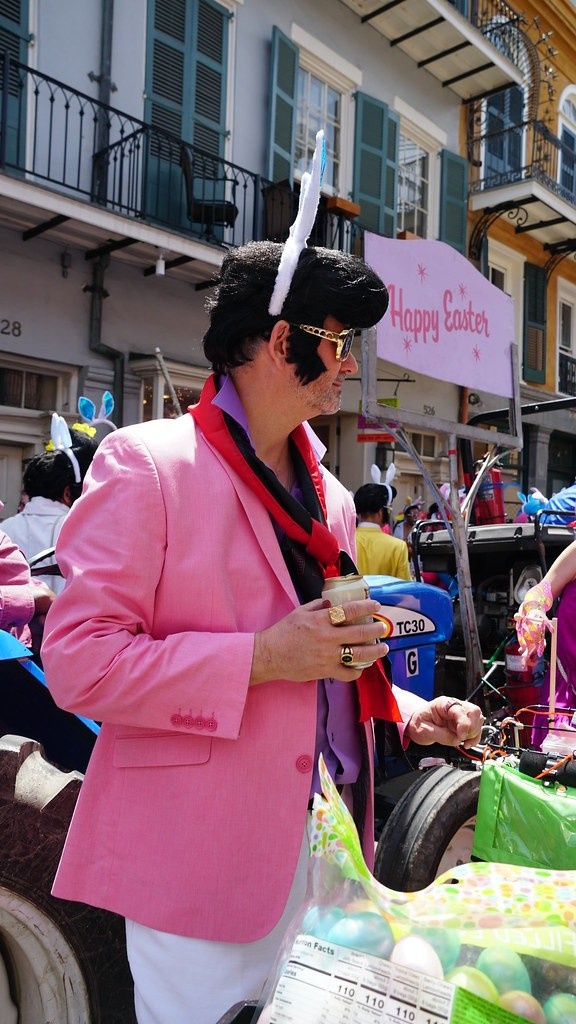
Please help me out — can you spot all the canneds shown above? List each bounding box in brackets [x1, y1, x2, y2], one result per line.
[321, 572, 377, 669]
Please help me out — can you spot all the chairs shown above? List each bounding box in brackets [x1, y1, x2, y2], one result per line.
[179, 146, 239, 244]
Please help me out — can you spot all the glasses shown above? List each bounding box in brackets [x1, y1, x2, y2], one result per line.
[384, 505, 393, 513]
[289, 322, 354, 363]
[408, 514, 420, 518]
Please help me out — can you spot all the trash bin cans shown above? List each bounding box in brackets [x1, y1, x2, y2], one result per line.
[358, 573, 454, 774]
[412, 508, 575, 710]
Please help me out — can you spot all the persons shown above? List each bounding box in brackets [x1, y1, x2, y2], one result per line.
[353, 483, 412, 582]
[392, 499, 459, 597]
[514, 536, 576, 747]
[41, 241, 485, 1024]
[1, 429, 100, 672]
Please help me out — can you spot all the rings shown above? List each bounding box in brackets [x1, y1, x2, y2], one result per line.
[443, 698, 463, 712]
[340, 647, 354, 664]
[328, 605, 347, 625]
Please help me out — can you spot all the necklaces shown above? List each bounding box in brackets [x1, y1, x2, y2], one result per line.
[286, 463, 293, 492]
